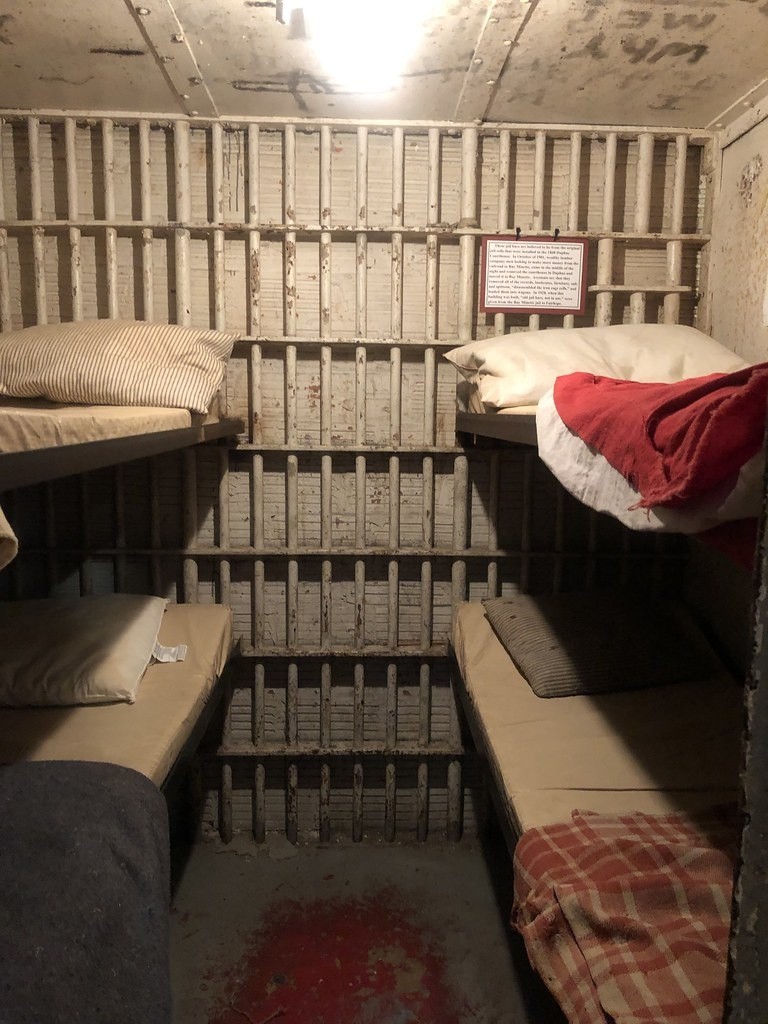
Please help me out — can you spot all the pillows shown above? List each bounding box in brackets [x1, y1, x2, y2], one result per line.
[0, 319, 241, 415]
[442, 324, 752, 408]
[0, 595, 188, 704]
[482, 594, 694, 698]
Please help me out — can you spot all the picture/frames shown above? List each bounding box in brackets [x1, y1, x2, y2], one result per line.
[479, 235, 588, 316]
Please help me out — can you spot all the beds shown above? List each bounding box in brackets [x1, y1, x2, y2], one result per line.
[0, 406, 245, 570]
[0, 604, 235, 1024]
[456, 372, 768, 532]
[450, 604, 768, 1024]
